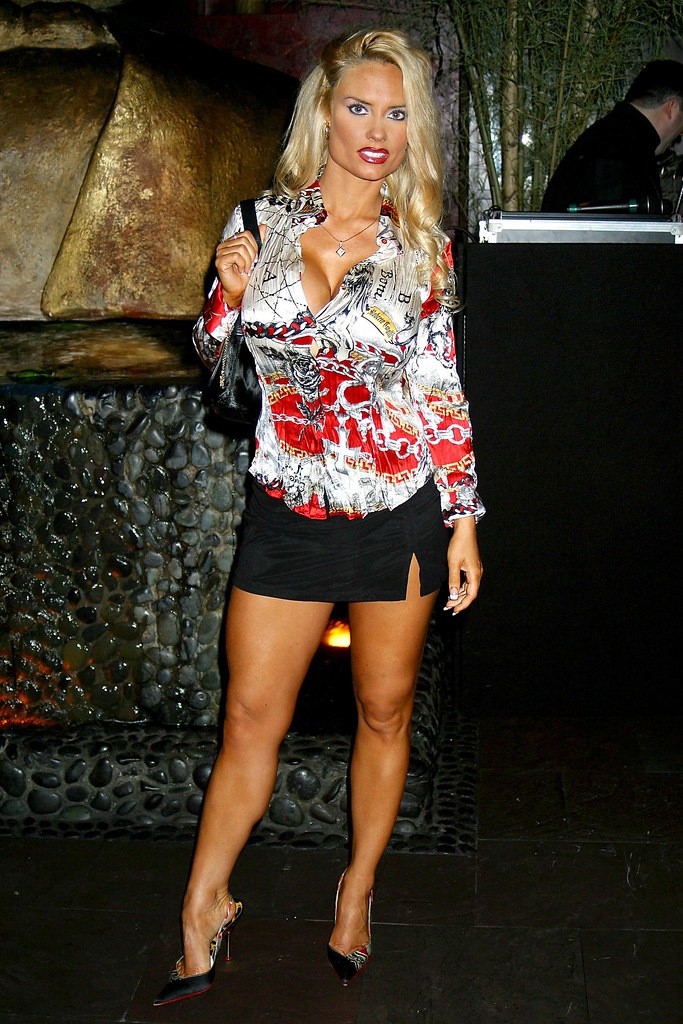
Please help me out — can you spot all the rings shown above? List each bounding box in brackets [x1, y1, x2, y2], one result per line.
[234, 235, 237, 239]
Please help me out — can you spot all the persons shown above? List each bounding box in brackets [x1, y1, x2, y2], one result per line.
[540, 58, 683, 221]
[152, 28, 487, 1009]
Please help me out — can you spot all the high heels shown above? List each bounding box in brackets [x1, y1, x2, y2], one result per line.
[152, 898, 242, 1006]
[326, 867, 373, 986]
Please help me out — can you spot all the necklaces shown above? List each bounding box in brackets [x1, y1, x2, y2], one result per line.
[319, 216, 378, 257]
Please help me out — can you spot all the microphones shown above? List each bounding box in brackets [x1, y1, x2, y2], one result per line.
[567, 195, 673, 215]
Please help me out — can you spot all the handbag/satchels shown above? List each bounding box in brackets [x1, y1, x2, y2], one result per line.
[200, 199, 264, 438]
